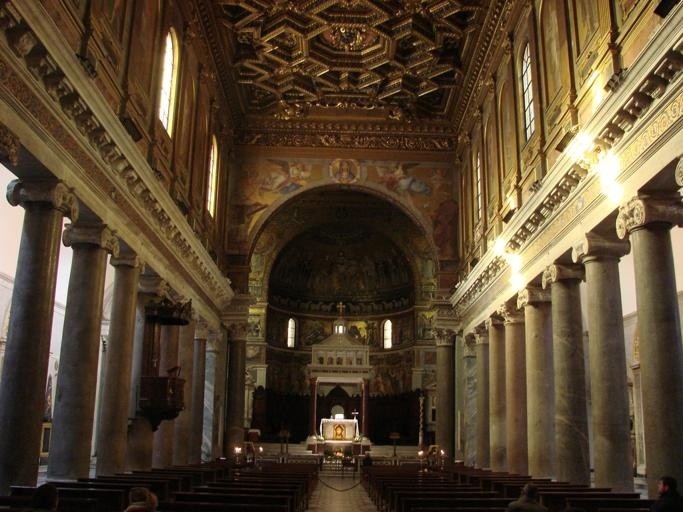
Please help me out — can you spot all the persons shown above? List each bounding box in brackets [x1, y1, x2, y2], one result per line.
[333, 424, 344, 440]
